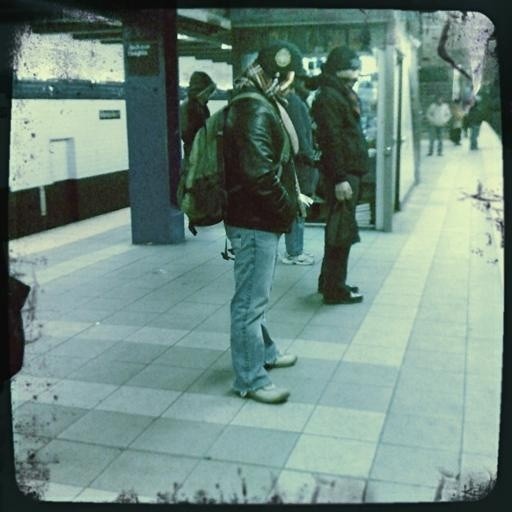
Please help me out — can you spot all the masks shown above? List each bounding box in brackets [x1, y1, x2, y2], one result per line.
[339, 77, 357, 87]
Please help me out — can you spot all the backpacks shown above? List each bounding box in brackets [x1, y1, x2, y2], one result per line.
[175, 92, 272, 225]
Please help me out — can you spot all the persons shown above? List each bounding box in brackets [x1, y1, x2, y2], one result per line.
[309, 42, 370, 306]
[178, 74, 216, 163]
[425, 86, 487, 157]
[216, 41, 314, 404]
[277, 69, 320, 267]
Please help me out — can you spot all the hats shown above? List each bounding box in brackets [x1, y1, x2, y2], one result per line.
[326, 47, 361, 71]
[257, 42, 306, 76]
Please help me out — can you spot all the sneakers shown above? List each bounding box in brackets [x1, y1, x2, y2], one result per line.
[324, 284, 363, 304]
[266, 353, 297, 367]
[281, 251, 315, 265]
[250, 383, 290, 403]
